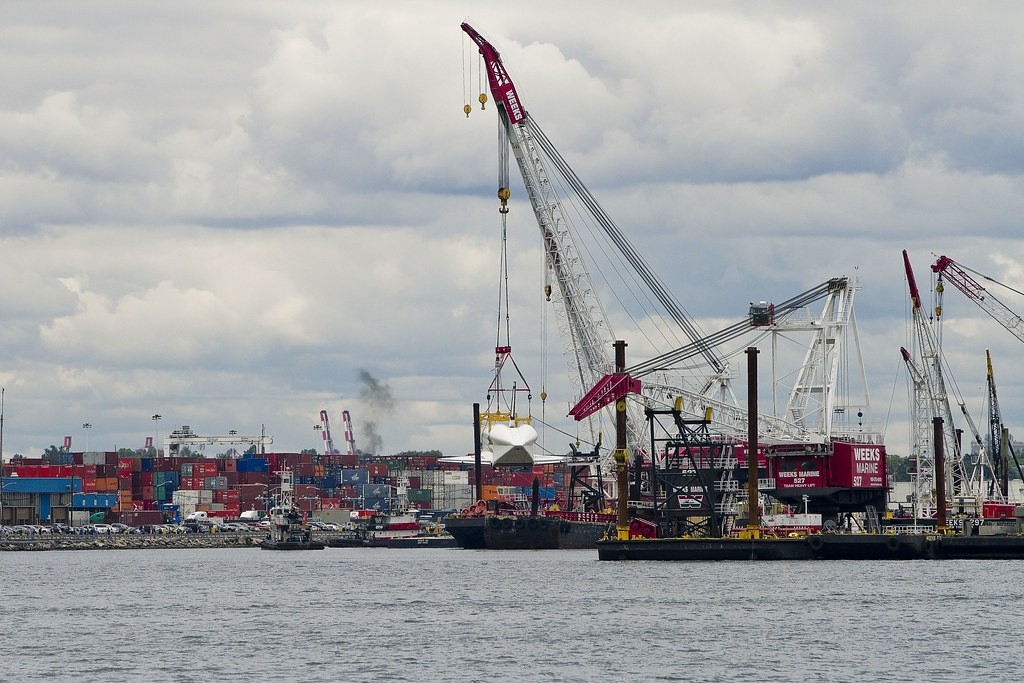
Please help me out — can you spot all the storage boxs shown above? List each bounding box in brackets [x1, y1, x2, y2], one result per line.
[0, 452, 616, 527]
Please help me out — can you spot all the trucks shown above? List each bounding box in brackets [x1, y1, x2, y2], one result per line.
[185, 510, 235, 523]
[239, 510, 266, 522]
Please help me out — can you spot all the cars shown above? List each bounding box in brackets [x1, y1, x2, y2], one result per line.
[0, 519, 447, 536]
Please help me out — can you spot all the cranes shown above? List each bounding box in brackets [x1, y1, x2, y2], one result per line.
[459, 18, 1023, 543]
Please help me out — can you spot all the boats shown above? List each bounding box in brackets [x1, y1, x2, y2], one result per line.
[481, 423, 538, 470]
[443, 515, 618, 550]
